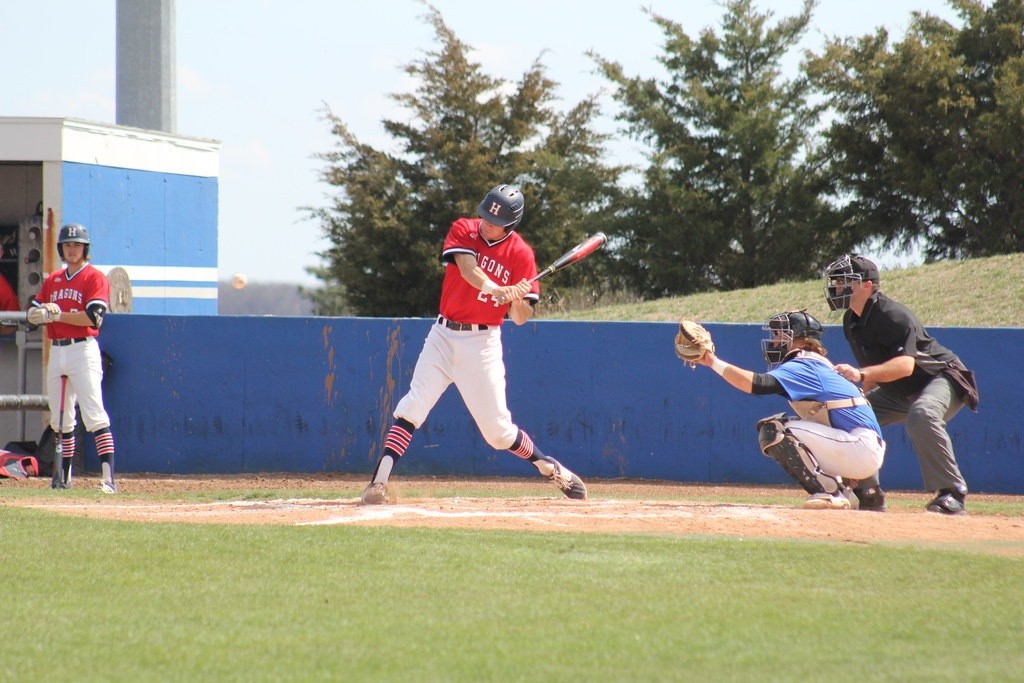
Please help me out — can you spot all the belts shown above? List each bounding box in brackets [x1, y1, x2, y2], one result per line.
[439, 316, 488, 331]
[52, 337, 86, 346]
[877, 435, 882, 447]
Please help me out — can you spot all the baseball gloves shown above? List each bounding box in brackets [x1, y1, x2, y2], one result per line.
[674, 320, 715, 363]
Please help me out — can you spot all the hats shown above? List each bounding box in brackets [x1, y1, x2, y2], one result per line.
[830, 256, 879, 284]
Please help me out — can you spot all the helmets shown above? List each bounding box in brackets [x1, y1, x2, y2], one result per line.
[477, 184, 525, 235]
[774, 312, 823, 340]
[57, 224, 90, 259]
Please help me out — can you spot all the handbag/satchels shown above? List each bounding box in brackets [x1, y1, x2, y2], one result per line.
[940, 357, 979, 414]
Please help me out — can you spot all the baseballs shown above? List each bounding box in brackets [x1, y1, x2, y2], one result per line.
[232, 274, 248, 289]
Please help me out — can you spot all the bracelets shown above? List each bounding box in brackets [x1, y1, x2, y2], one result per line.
[859, 368, 865, 383]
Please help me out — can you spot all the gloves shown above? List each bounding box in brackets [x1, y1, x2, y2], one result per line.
[28, 300, 62, 325]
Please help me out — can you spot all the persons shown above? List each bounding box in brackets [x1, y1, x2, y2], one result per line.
[822, 255, 980, 515]
[364, 187, 586, 504]
[25, 225, 117, 493]
[0, 243, 20, 334]
[675, 309, 886, 507]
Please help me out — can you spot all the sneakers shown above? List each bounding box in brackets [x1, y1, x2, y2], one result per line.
[363, 482, 386, 504]
[804, 491, 851, 510]
[49, 482, 73, 490]
[94, 481, 116, 494]
[546, 456, 587, 500]
[853, 488, 886, 512]
[926, 486, 968, 515]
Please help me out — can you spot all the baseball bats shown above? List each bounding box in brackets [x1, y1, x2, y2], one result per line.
[51, 375, 68, 488]
[497, 233, 609, 305]
